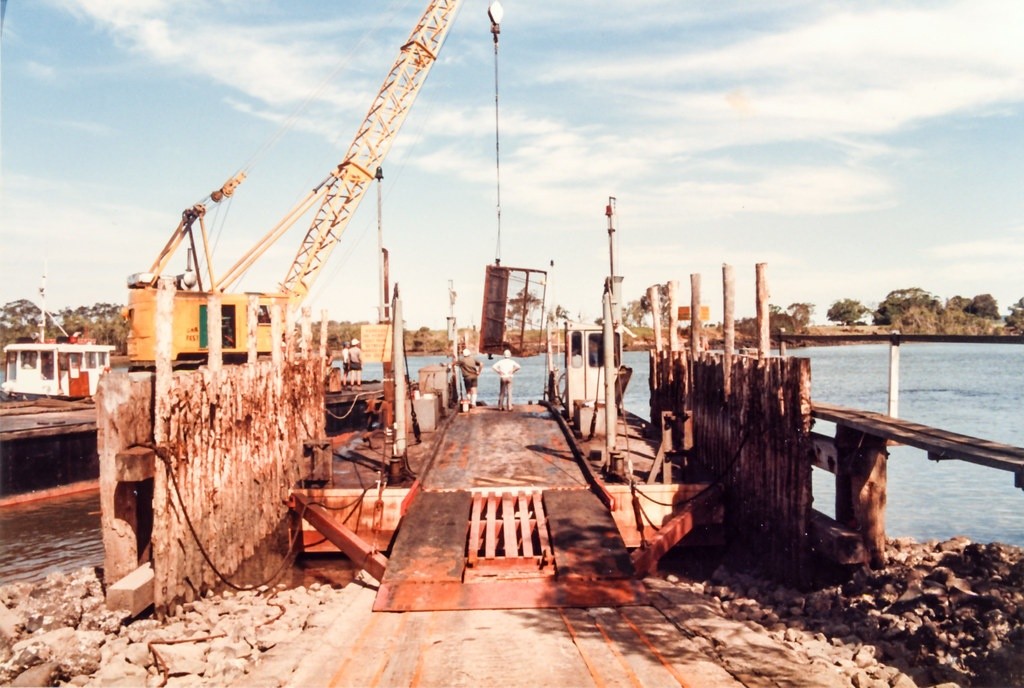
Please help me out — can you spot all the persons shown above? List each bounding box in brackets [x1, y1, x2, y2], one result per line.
[493, 350, 520, 412]
[325, 344, 333, 375]
[341, 339, 362, 387]
[453, 349, 483, 408]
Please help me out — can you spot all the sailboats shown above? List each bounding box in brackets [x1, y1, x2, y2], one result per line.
[0, 265, 117, 399]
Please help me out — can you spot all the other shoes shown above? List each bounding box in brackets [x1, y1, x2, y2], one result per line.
[470, 404, 475, 409]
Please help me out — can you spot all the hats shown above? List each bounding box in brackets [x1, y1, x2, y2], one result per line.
[504, 350, 511, 357]
[342, 341, 350, 346]
[463, 349, 470, 356]
[351, 339, 359, 345]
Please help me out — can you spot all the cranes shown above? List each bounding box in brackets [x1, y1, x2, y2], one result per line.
[124, 0, 503, 370]
